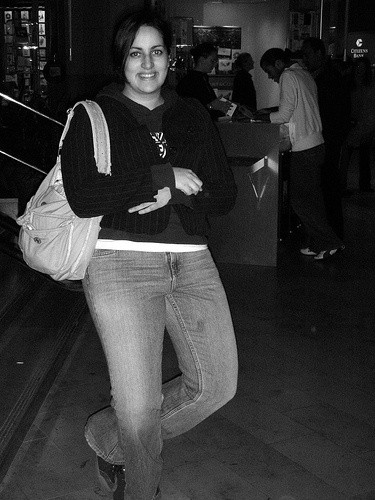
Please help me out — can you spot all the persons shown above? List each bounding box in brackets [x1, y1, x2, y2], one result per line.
[176, 42, 234, 122]
[253, 46, 346, 261]
[230, 52, 256, 114]
[59, 8, 238, 500]
[256, 35, 345, 243]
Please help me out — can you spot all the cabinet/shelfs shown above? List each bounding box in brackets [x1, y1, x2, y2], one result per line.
[0, 0, 65, 112]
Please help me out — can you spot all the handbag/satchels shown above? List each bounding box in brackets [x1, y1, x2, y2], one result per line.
[232, 102, 257, 120]
[14, 98, 112, 282]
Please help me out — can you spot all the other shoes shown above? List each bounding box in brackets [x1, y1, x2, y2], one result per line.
[300, 247, 320, 255]
[313, 243, 346, 260]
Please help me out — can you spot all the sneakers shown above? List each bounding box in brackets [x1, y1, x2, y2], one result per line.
[94, 452, 125, 496]
